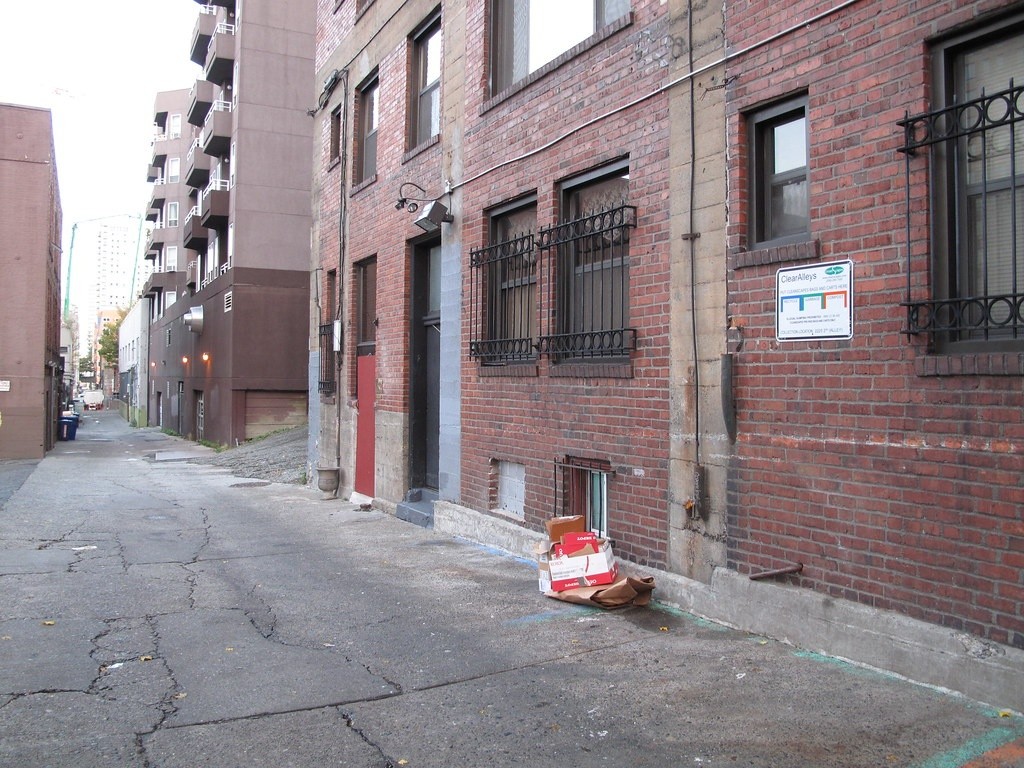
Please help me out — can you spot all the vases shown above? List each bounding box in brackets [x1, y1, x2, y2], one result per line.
[316, 466, 341, 500]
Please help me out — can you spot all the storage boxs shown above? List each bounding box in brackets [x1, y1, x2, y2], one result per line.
[533, 543, 551, 592]
[549, 544, 619, 591]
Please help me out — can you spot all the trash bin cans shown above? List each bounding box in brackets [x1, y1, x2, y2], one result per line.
[59, 411, 81, 441]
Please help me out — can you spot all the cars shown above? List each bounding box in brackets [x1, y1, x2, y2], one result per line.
[82, 391, 105, 410]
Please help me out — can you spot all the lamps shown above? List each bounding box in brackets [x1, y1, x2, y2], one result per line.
[395, 182, 426, 211]
[413, 201, 454, 233]
